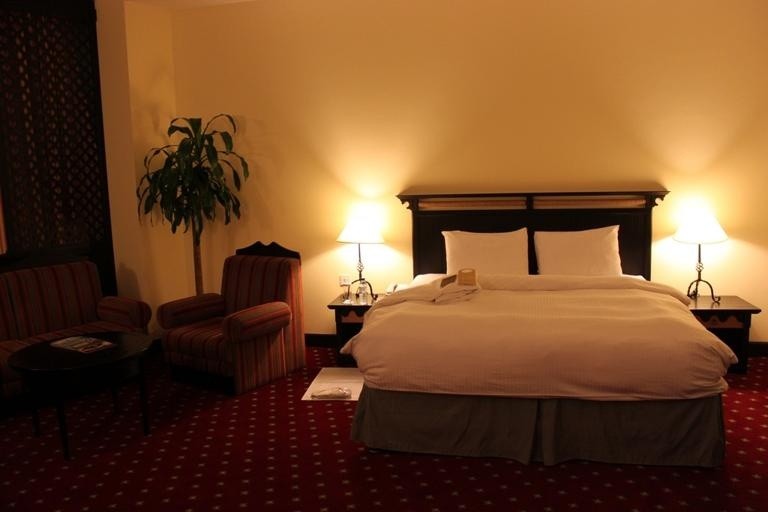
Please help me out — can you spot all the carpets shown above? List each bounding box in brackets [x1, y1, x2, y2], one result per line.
[300, 364, 366, 403]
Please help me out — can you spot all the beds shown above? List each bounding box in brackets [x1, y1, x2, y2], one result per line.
[344, 189, 727, 468]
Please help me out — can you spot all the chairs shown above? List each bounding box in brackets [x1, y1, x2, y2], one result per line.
[156, 251, 306, 395]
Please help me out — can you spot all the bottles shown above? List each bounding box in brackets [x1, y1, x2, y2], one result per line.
[359, 278, 373, 306]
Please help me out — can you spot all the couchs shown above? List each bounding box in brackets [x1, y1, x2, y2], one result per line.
[1, 254, 151, 423]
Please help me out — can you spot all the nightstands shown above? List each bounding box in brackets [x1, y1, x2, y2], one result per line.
[688, 294, 760, 374]
[326, 290, 391, 367]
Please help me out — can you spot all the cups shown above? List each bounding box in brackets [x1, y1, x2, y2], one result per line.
[338, 276, 351, 303]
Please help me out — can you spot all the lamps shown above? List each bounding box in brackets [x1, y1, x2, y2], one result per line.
[333, 201, 387, 300]
[675, 203, 730, 304]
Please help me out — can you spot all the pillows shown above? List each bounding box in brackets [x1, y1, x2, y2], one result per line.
[434, 226, 624, 281]
